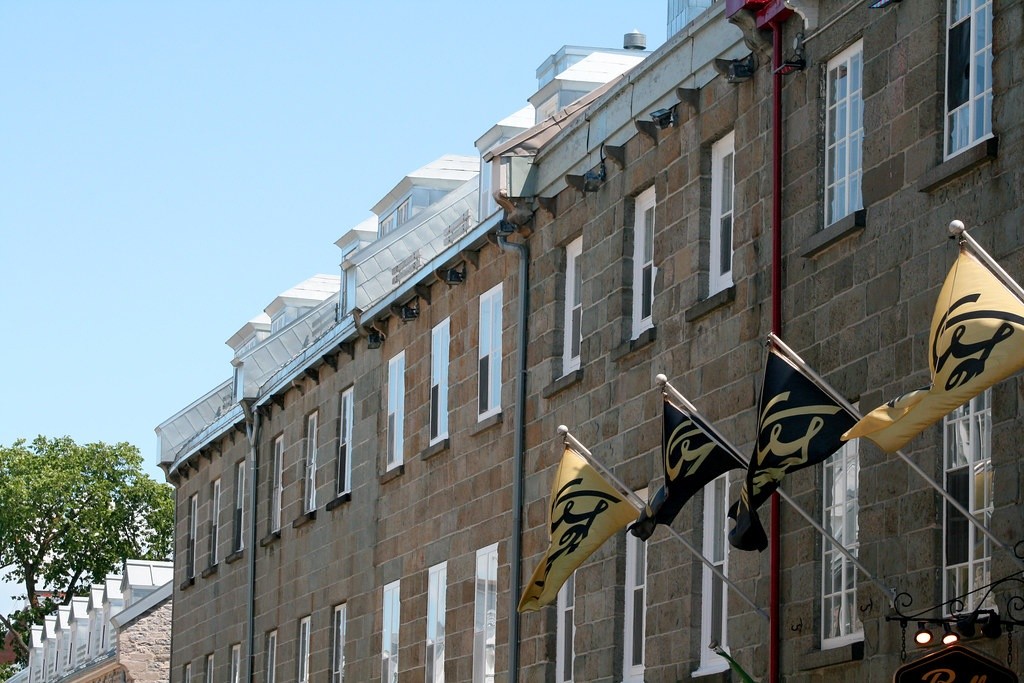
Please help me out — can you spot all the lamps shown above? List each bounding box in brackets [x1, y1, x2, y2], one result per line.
[446, 266, 466, 286]
[914, 608, 1003, 647]
[581, 169, 600, 193]
[648, 108, 676, 129]
[367, 332, 384, 349]
[494, 219, 518, 236]
[772, 54, 806, 77]
[726, 61, 754, 84]
[401, 305, 419, 322]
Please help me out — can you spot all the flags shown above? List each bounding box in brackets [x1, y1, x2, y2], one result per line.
[727, 349, 859, 551]
[517, 447, 641, 611]
[840, 246, 1024, 454]
[626, 397, 747, 541]
